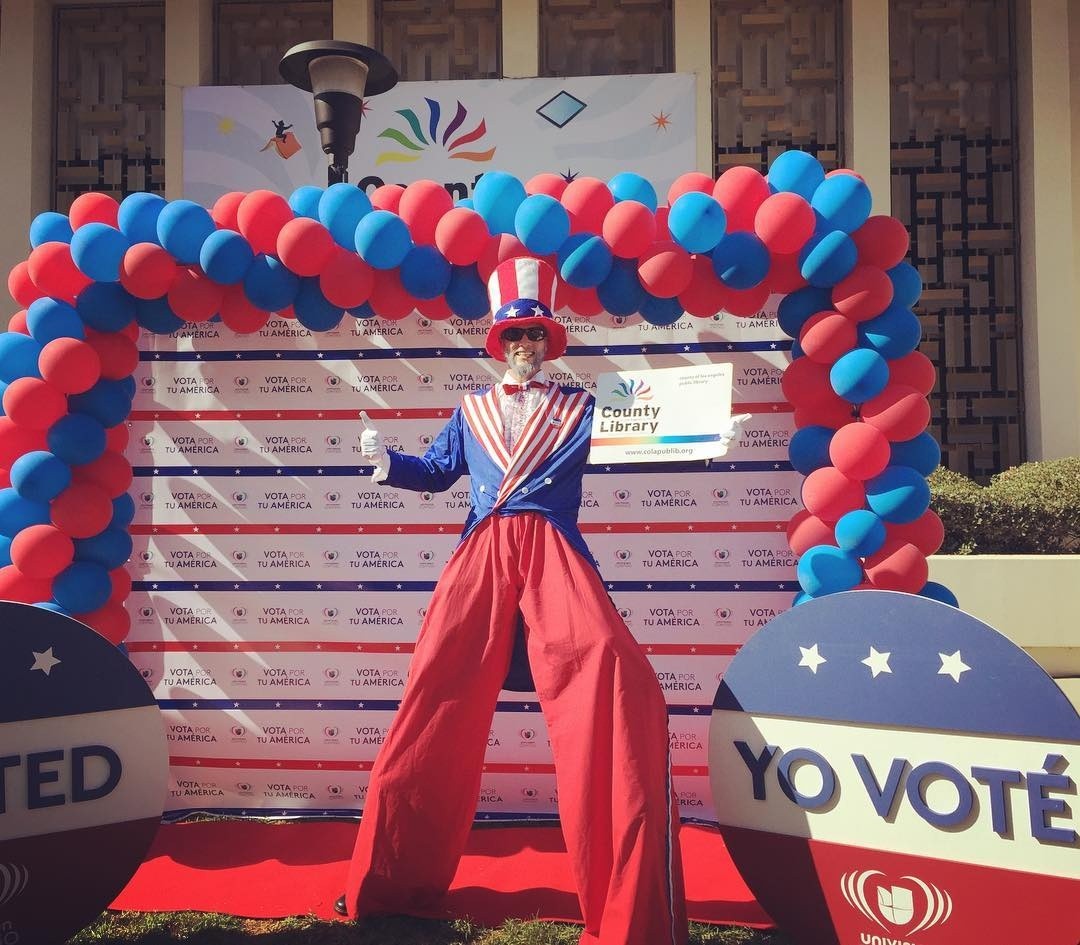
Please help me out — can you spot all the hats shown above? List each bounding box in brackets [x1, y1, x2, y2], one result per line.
[486, 255, 568, 363]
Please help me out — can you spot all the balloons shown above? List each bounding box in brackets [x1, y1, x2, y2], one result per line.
[3, 147, 968, 638]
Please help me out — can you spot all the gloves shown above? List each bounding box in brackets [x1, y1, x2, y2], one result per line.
[359, 410, 391, 484]
[719, 412, 753, 449]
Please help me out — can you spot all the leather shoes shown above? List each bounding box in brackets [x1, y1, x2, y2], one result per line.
[334, 892, 348, 915]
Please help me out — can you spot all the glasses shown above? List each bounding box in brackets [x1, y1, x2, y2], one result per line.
[500, 327, 546, 341]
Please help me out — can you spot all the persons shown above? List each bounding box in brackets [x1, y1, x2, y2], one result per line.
[358, 259, 750, 944]
